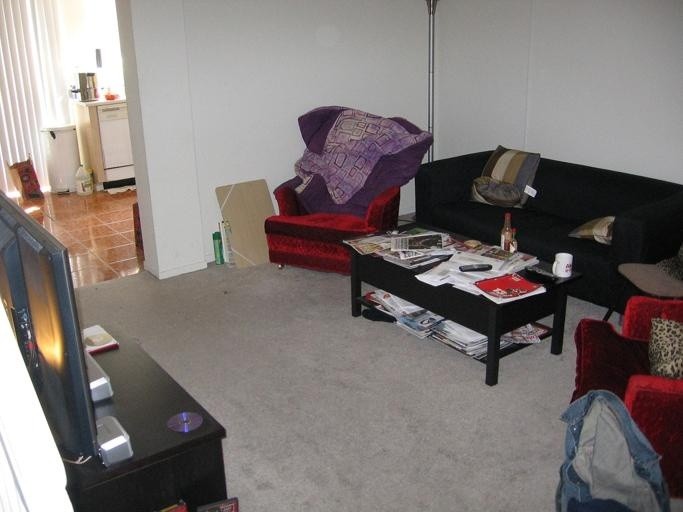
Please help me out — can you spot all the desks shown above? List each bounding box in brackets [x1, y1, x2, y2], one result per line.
[340, 223, 584, 386]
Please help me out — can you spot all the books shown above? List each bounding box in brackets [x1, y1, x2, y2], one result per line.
[342, 223, 548, 360]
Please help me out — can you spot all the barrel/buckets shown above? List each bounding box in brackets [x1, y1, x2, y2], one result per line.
[76, 164, 93, 196]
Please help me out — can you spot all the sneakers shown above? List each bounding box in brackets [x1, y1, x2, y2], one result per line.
[362, 308, 395, 322]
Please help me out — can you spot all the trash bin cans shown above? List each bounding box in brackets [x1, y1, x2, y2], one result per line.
[39, 123, 81, 193]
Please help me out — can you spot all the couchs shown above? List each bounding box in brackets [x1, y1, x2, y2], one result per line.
[414, 149, 683, 322]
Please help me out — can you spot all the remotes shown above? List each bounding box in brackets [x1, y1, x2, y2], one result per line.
[459, 264, 492, 272]
[524, 265, 557, 282]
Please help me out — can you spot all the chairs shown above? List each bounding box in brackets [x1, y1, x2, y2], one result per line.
[264, 106, 433, 277]
[567, 296, 683, 501]
[601, 240, 683, 321]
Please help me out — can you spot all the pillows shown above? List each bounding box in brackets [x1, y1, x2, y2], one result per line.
[647, 317, 682, 378]
[567, 216, 616, 246]
[472, 146, 541, 210]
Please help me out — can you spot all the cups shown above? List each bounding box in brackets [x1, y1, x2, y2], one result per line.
[552, 253, 573, 278]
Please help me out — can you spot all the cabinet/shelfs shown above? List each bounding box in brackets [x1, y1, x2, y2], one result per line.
[89, 104, 135, 190]
[65, 325, 227, 512]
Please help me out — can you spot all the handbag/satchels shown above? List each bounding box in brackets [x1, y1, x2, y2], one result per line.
[4, 153, 44, 203]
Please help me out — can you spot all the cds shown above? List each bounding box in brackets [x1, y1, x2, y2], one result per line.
[167, 411, 203, 434]
[86, 333, 112, 346]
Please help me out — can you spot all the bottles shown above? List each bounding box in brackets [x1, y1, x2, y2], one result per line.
[500, 213, 512, 251]
[509, 228, 518, 253]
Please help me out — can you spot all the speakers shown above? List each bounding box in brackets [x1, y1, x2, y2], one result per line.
[94, 416, 135, 468]
[82, 349, 114, 402]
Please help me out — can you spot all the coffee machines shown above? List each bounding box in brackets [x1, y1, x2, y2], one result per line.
[78, 72, 101, 102]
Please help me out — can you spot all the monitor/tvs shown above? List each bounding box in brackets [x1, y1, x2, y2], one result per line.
[0, 191, 100, 487]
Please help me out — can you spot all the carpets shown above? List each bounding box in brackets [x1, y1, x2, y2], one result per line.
[74, 211, 681, 511]
